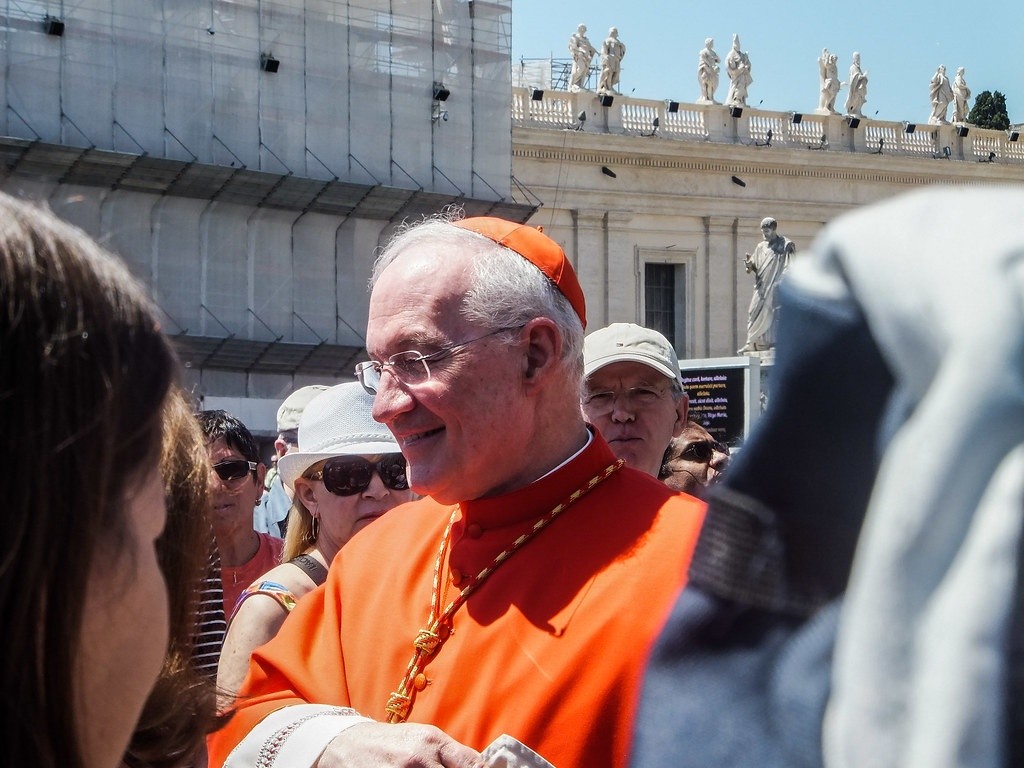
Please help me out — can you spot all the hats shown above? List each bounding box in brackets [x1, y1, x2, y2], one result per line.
[449, 217, 587, 333]
[580, 322, 684, 393]
[276, 385, 332, 433]
[277, 381, 401, 492]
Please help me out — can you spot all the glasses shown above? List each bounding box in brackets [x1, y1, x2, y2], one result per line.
[662, 441, 730, 466]
[212, 460, 258, 480]
[303, 454, 410, 497]
[354, 325, 526, 395]
[581, 386, 675, 416]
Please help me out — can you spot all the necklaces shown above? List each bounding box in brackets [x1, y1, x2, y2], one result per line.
[375, 456, 626, 724]
[230, 566, 239, 583]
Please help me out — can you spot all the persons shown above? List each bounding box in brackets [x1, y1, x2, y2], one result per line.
[698, 38, 721, 100]
[928, 64, 954, 125]
[0, 191, 1024, 768]
[596, 28, 626, 94]
[726, 34, 753, 105]
[737, 218, 795, 354]
[567, 25, 597, 91]
[952, 67, 971, 123]
[844, 52, 869, 114]
[817, 50, 841, 111]
